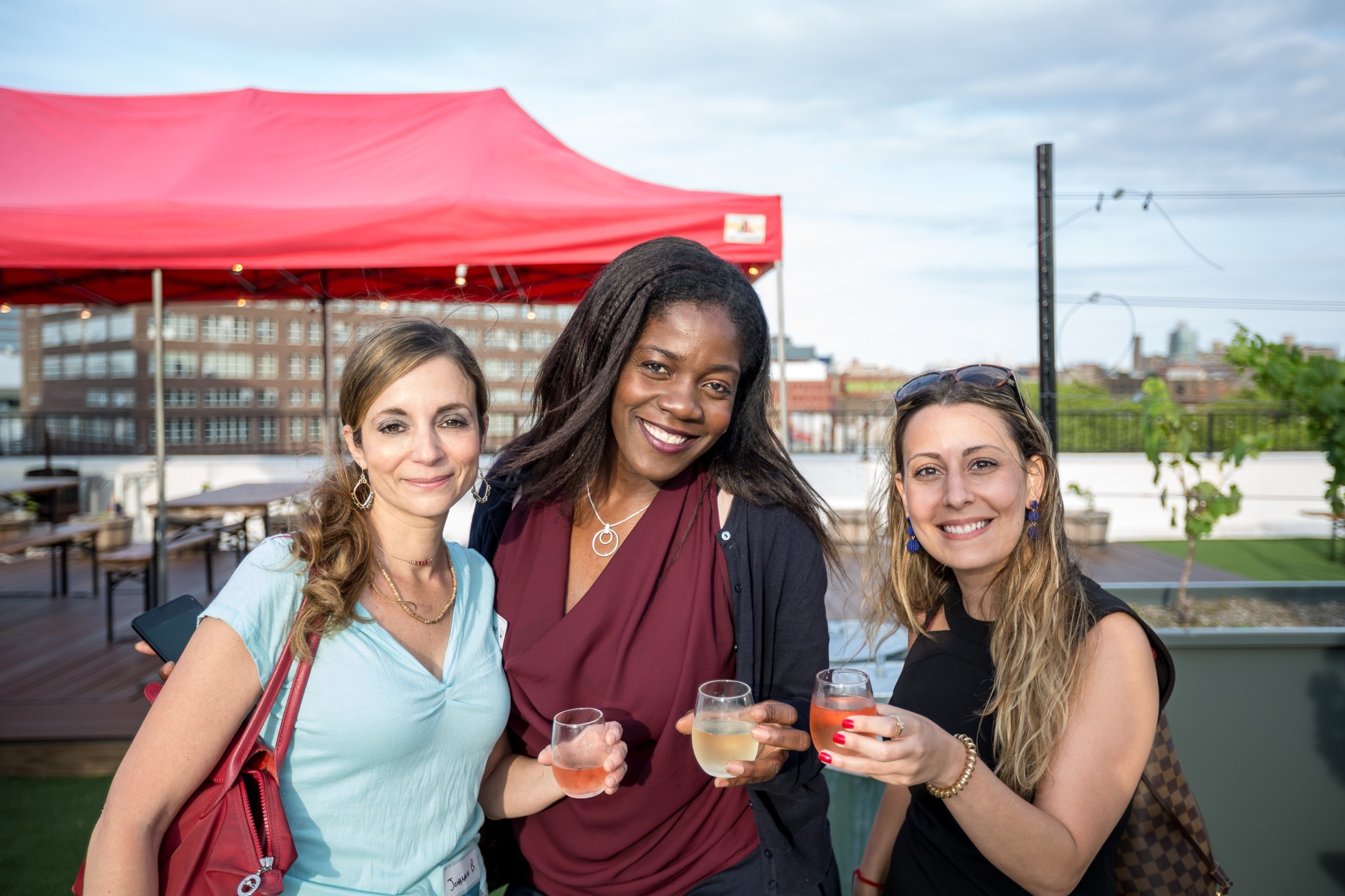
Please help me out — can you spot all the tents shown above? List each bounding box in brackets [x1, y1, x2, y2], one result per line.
[0, 88, 787, 606]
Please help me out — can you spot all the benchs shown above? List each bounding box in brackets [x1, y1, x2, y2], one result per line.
[0, 516, 248, 647]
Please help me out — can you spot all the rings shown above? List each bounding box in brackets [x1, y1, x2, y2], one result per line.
[888, 715, 905, 739]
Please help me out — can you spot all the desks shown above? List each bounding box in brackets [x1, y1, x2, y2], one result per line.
[146, 483, 315, 612]
[0, 475, 87, 599]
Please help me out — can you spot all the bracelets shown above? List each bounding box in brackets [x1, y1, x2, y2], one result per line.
[852, 868, 885, 889]
[926, 733, 977, 799]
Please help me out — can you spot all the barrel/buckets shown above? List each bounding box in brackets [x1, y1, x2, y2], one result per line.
[68, 515, 133, 556]
[826, 509, 878, 555]
[1063, 511, 1109, 545]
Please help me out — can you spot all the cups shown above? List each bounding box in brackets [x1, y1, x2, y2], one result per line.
[809, 668, 877, 757]
[691, 679, 760, 778]
[551, 708, 612, 799]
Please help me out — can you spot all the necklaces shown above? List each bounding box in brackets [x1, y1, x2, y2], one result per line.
[373, 539, 457, 624]
[371, 538, 442, 566]
[585, 467, 649, 557]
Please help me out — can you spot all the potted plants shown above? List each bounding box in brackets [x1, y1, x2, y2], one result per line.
[1059, 484, 1110, 547]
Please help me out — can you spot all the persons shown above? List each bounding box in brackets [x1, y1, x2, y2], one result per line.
[82, 301, 628, 896]
[851, 368, 1176, 896]
[468, 233, 861, 896]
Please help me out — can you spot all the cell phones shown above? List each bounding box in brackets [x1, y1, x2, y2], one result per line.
[131, 594, 205, 665]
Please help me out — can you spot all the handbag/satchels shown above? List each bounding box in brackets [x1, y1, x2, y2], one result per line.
[71, 533, 333, 896]
[1114, 704, 1233, 896]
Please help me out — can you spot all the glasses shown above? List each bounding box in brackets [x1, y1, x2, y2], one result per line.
[894, 364, 1028, 423]
